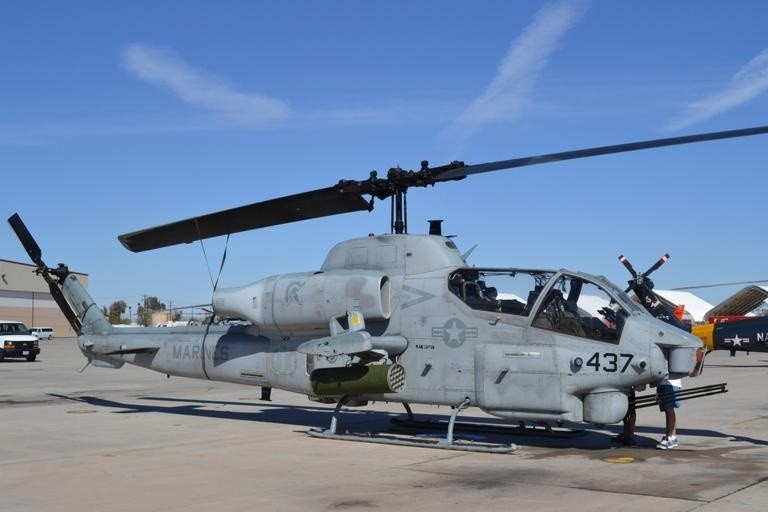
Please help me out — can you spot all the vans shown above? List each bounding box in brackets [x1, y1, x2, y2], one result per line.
[28, 326, 54, 340]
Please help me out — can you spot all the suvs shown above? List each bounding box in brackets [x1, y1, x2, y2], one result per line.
[0, 320, 41, 364]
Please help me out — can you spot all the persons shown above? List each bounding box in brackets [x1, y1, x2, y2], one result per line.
[611, 392, 638, 448]
[654, 375, 683, 451]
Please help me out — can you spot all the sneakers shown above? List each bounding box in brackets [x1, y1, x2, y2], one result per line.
[610, 432, 625, 442]
[656, 436, 679, 450]
[623, 438, 637, 447]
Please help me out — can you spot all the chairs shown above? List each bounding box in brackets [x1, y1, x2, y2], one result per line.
[462, 281, 493, 307]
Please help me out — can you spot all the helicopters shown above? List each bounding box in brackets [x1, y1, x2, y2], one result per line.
[6, 126, 768, 456]
[610, 249, 767, 355]
